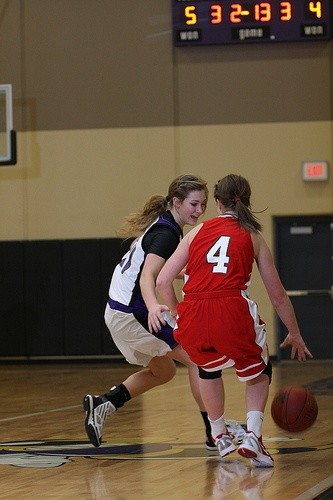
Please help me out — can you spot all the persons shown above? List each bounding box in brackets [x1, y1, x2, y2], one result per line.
[156, 175, 313, 467]
[83, 173, 249, 450]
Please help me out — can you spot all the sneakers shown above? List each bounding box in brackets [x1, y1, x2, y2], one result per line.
[237, 431, 274, 467]
[211, 426, 237, 457]
[83, 395, 116, 448]
[205, 424, 247, 450]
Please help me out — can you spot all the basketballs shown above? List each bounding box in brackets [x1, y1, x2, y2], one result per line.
[271, 385, 318, 433]
